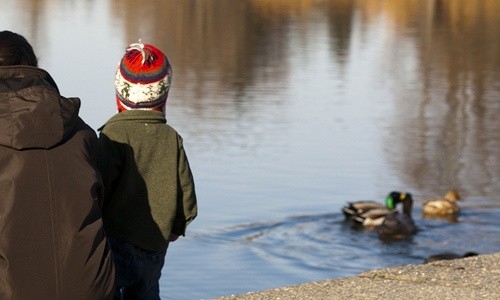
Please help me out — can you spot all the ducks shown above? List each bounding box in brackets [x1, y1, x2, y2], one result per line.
[342, 191, 418, 239]
[422, 189, 463, 214]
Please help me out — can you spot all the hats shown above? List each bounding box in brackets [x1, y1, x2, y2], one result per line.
[114, 38, 172, 112]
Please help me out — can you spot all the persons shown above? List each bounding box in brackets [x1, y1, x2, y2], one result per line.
[96, 39, 198, 300]
[0, 30, 122, 300]
